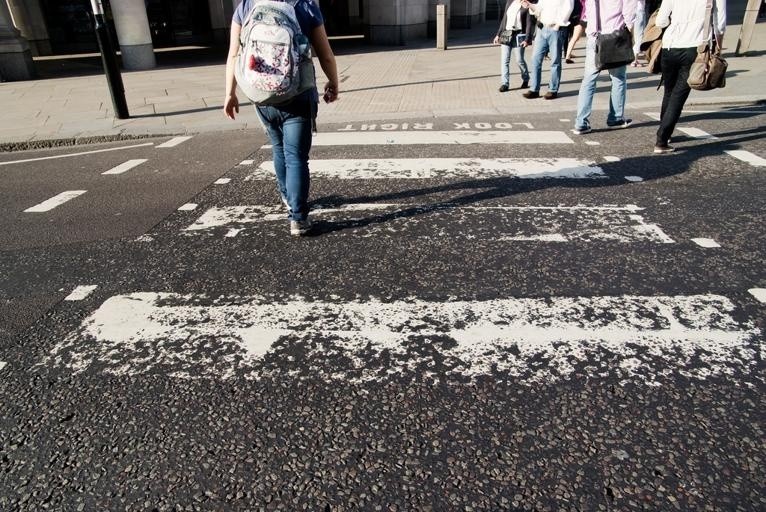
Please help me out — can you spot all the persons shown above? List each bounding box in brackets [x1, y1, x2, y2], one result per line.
[492, 0, 726, 153]
[224, 0, 339, 235]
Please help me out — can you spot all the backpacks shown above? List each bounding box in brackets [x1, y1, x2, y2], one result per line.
[231, 1, 306, 111]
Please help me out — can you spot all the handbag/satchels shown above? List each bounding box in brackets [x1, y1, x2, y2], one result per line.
[689, 1, 730, 93]
[496, 26, 518, 49]
[593, 25, 641, 74]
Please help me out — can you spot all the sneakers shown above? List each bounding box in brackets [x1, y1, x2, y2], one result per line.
[571, 124, 593, 137]
[564, 58, 575, 66]
[495, 82, 511, 94]
[519, 77, 530, 90]
[652, 142, 678, 155]
[522, 90, 540, 101]
[604, 117, 633, 129]
[543, 88, 560, 101]
[286, 216, 316, 241]
